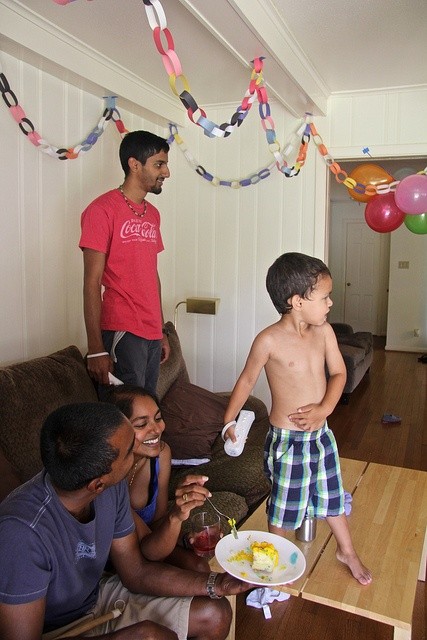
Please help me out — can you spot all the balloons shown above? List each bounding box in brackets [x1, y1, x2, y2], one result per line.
[347, 163, 396, 202]
[365, 192, 405, 234]
[404, 213, 427, 234]
[394, 174, 427, 214]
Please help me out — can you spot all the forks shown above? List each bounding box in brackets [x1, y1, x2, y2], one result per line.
[200, 493, 240, 541]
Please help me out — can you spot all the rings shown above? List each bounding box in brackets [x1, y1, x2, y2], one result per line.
[182, 493, 187, 501]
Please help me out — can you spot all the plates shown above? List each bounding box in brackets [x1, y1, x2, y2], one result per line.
[214, 528, 310, 589]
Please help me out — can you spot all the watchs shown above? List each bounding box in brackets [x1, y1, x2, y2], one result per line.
[207, 572, 223, 600]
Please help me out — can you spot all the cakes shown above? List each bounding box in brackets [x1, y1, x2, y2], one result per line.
[252, 540, 280, 573]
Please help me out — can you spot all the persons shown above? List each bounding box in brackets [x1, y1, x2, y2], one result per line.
[79, 130, 170, 398]
[0, 403, 293, 640]
[220, 253, 372, 585]
[103, 384, 212, 573]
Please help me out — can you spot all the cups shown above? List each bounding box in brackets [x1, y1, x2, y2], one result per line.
[189, 510, 222, 560]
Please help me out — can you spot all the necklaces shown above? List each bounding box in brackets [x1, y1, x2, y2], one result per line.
[119, 185, 147, 217]
[129, 463, 137, 486]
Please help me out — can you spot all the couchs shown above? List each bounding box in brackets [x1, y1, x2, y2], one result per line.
[2, 344, 249, 640]
[83, 321, 273, 507]
[329, 322, 374, 406]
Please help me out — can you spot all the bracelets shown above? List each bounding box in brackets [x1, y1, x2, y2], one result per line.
[221, 420, 237, 442]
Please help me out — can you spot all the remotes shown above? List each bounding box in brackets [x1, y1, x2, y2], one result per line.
[225, 410, 255, 456]
